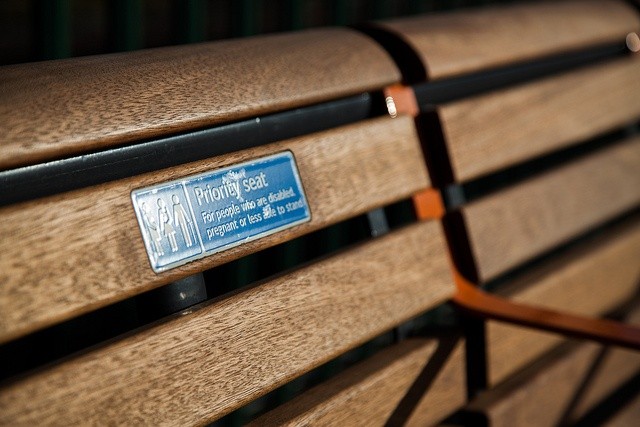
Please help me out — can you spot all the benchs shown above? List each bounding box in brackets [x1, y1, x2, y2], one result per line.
[0, 32, 638, 427]
[369, 2, 640, 427]
